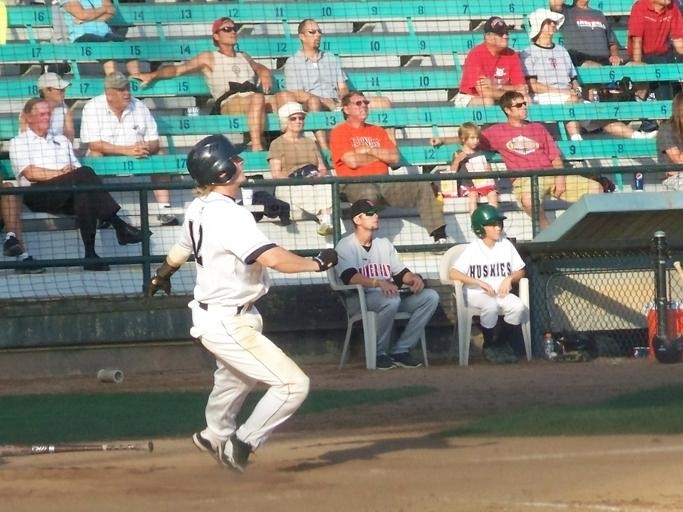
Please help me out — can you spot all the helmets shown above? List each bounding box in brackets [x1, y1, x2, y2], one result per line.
[186, 133, 248, 185]
[653, 333, 682, 364]
[471, 205, 507, 239]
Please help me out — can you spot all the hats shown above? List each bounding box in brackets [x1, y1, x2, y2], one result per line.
[37, 72, 72, 90]
[528, 9, 566, 40]
[350, 199, 385, 218]
[484, 16, 514, 33]
[104, 71, 130, 88]
[278, 102, 307, 121]
[212, 17, 230, 47]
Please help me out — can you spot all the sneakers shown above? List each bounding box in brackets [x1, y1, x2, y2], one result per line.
[2, 236, 26, 256]
[191, 427, 225, 466]
[218, 428, 251, 473]
[16, 256, 47, 275]
[376, 355, 397, 370]
[641, 130, 659, 138]
[431, 236, 456, 254]
[388, 352, 423, 368]
[316, 221, 334, 236]
[156, 205, 179, 226]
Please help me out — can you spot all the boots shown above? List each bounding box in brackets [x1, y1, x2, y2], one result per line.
[481, 318, 521, 363]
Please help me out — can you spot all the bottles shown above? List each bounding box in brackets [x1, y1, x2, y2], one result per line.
[544, 332, 554, 359]
[593, 91, 599, 102]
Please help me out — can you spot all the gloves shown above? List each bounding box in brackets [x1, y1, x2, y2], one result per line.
[149, 270, 171, 299]
[314, 249, 338, 272]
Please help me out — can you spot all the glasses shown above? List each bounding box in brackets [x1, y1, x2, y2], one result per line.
[306, 29, 322, 34]
[215, 25, 239, 32]
[351, 100, 369, 106]
[288, 117, 306, 120]
[543, 21, 557, 25]
[512, 102, 526, 108]
[366, 209, 377, 216]
[497, 32, 509, 37]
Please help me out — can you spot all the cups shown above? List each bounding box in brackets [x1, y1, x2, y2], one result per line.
[633, 172, 645, 190]
[241, 180, 253, 203]
[96, 370, 124, 382]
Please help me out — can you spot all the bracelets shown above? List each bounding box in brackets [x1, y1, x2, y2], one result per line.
[372, 277, 378, 288]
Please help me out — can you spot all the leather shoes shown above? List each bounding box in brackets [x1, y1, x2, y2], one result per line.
[117, 223, 153, 246]
[84, 253, 110, 271]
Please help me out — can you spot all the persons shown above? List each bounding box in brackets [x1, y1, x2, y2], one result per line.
[449, 92, 603, 231]
[265, 94, 448, 255]
[131, 15, 398, 150]
[656, 94, 682, 191]
[550, 1, 682, 131]
[333, 198, 441, 371]
[149, 134, 339, 475]
[448, 205, 527, 365]
[456, 9, 659, 140]
[0, 0, 177, 274]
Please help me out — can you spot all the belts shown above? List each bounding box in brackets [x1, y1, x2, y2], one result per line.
[199, 302, 253, 316]
